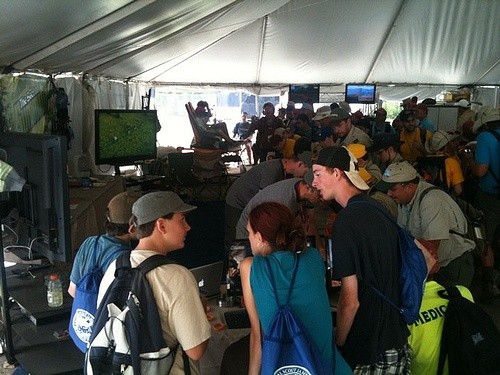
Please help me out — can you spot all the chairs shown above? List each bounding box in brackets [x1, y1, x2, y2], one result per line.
[186, 102, 251, 167]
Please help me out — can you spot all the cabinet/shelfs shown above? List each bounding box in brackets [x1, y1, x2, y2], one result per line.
[0, 247, 84, 375]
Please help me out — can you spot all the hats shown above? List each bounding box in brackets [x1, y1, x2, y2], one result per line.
[313, 145, 370, 191]
[399, 110, 414, 121]
[297, 151, 312, 168]
[367, 133, 401, 153]
[329, 109, 349, 122]
[274, 127, 286, 138]
[347, 143, 367, 160]
[312, 106, 332, 121]
[374, 108, 387, 116]
[288, 100, 295, 106]
[302, 168, 314, 185]
[351, 111, 363, 119]
[424, 98, 500, 153]
[375, 161, 418, 190]
[105, 192, 138, 224]
[131, 191, 198, 226]
[302, 103, 313, 111]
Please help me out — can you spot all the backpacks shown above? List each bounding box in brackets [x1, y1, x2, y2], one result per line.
[346, 201, 428, 323]
[67, 234, 134, 353]
[83, 248, 182, 375]
[435, 279, 500, 375]
[418, 155, 454, 195]
[257, 253, 330, 375]
[417, 186, 488, 259]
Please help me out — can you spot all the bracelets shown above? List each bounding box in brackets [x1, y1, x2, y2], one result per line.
[335, 339, 343, 351]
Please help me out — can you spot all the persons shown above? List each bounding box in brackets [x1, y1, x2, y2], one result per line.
[69, 96, 500, 375]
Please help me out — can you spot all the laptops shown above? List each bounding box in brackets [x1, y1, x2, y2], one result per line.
[189, 261, 224, 301]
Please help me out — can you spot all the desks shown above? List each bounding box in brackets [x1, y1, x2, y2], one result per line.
[71, 176, 123, 251]
[168, 281, 342, 375]
[126, 176, 165, 192]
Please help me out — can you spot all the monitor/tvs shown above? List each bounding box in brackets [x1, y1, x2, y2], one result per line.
[95, 108, 157, 173]
[0, 131, 72, 262]
[289, 84, 319, 103]
[345, 84, 376, 104]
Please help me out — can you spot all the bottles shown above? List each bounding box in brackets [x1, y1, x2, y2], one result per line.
[47, 274, 63, 307]
